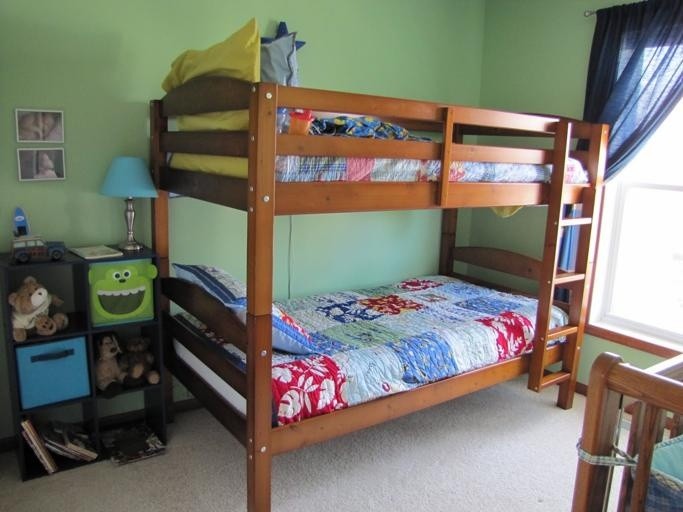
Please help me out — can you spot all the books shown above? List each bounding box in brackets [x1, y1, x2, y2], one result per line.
[20, 415, 166, 475]
[67, 244, 124, 260]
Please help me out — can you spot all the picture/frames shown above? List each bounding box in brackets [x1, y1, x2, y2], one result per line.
[15, 106, 63, 185]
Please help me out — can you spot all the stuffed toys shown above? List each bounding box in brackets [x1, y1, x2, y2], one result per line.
[94, 331, 160, 398]
[7, 276, 69, 343]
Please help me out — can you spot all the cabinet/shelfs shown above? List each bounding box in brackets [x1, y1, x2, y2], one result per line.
[1, 243, 170, 482]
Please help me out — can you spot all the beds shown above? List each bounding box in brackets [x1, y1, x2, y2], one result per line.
[149, 74, 681, 512]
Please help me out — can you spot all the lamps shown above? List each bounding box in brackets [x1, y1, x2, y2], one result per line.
[99, 156, 155, 253]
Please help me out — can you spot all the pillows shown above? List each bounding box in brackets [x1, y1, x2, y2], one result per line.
[157, 18, 305, 178]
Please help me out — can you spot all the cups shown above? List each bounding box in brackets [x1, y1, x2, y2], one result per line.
[288, 108, 312, 137]
